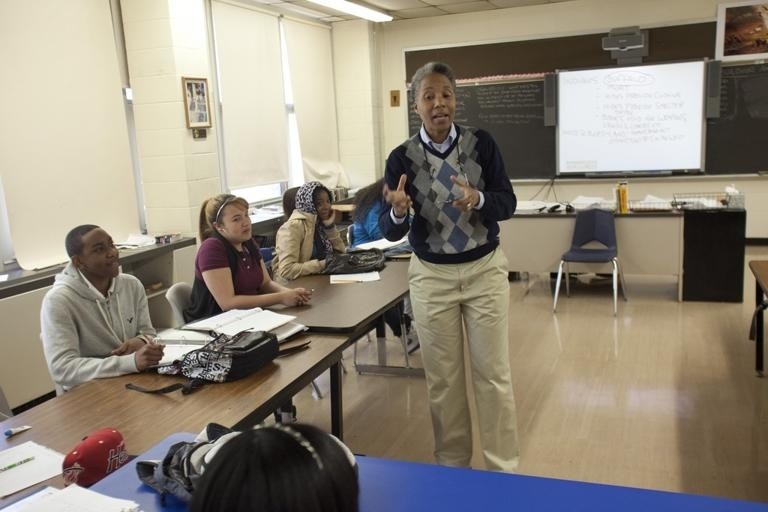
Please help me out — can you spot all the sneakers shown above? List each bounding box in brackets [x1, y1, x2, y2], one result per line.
[401, 326, 421, 355]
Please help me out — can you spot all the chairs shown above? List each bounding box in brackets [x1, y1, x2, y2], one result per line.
[552, 208, 628, 316]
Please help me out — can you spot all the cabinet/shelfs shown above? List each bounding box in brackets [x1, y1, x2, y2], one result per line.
[247, 202, 291, 233]
[0, 233, 196, 410]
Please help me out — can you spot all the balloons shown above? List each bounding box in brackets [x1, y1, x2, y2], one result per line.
[113, 233, 182, 249]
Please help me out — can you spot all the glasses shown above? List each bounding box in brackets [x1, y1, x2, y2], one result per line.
[428, 166, 470, 207]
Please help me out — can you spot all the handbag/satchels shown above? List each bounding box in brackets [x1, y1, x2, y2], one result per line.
[175, 326, 282, 385]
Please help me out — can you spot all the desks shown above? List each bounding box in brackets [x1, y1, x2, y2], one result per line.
[748, 260, 767, 378]
[682, 209, 746, 302]
[238, 261, 427, 378]
[88, 431, 767, 512]
[498, 211, 686, 302]
[0, 309, 352, 512]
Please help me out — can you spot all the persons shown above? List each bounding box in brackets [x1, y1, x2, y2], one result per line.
[186, 422, 359, 512]
[348, 178, 427, 354]
[379, 61, 519, 476]
[183, 192, 313, 423]
[271, 181, 347, 275]
[40, 224, 165, 396]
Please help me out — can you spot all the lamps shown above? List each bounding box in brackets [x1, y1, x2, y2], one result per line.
[308, 0, 394, 23]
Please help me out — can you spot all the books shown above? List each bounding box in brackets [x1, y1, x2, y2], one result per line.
[0, 441, 139, 512]
[630, 195, 728, 212]
[512, 179, 621, 214]
[147, 307, 309, 369]
[355, 237, 412, 253]
[250, 232, 275, 265]
[329, 270, 381, 284]
[327, 188, 347, 202]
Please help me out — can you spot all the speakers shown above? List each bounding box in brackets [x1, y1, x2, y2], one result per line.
[600, 34, 645, 52]
[706, 60, 722, 120]
[543, 74, 555, 126]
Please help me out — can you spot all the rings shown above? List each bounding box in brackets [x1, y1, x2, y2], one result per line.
[467, 203, 472, 210]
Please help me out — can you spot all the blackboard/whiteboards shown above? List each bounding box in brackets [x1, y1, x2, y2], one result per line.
[407, 52, 768, 185]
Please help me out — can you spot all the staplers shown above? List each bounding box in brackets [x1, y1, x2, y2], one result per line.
[547, 205, 562, 213]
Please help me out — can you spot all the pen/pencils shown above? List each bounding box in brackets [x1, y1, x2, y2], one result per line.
[0, 457, 36, 472]
[140, 330, 165, 356]
[333, 279, 363, 283]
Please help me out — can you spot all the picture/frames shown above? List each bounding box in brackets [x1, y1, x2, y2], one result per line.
[714, 0, 768, 64]
[181, 77, 213, 130]
